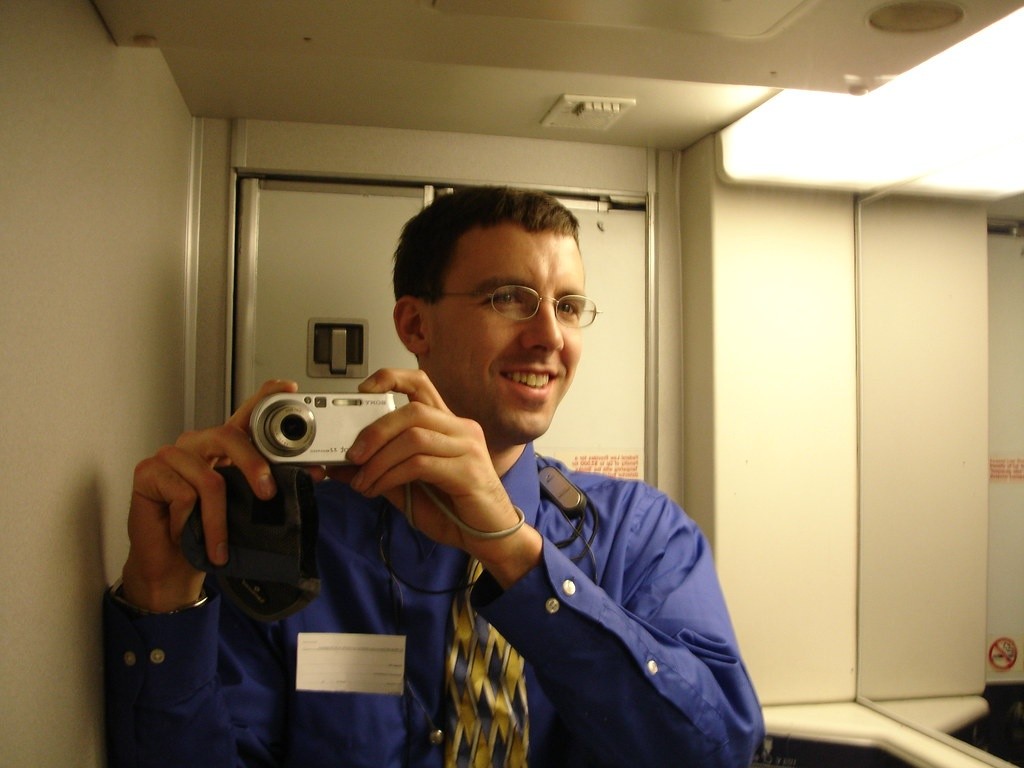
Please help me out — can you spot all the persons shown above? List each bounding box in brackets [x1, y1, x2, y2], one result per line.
[101, 186, 765, 768]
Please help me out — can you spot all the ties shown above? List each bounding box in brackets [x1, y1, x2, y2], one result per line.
[439, 554, 531, 768]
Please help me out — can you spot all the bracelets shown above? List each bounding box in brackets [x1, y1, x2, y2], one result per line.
[110, 576, 208, 616]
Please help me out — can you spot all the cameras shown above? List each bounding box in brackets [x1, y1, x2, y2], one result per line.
[248, 393, 394, 466]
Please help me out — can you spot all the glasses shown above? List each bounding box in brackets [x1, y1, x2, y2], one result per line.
[431, 285, 604, 329]
